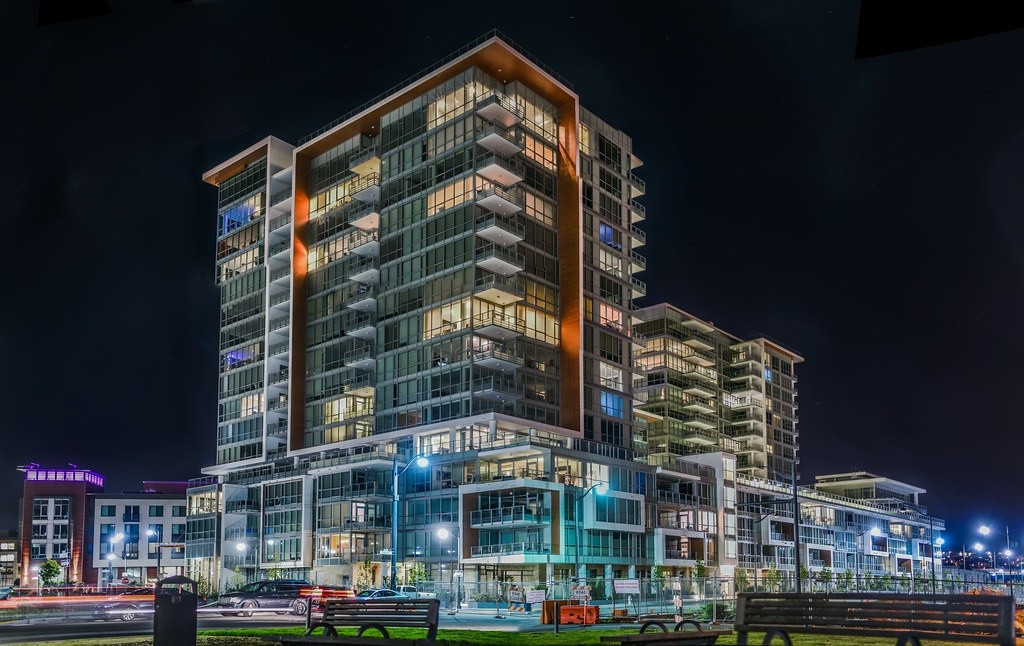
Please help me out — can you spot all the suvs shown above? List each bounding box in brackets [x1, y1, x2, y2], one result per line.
[215, 579, 320, 618]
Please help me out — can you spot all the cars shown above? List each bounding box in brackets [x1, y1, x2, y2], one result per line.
[346, 588, 415, 612]
[93, 587, 211, 622]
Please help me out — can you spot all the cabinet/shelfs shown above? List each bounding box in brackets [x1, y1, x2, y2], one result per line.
[560, 605, 600, 625]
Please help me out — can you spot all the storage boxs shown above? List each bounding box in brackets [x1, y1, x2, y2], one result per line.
[615, 610, 628, 616]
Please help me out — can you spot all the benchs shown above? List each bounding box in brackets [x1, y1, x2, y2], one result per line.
[735, 591, 1017, 646]
[261, 599, 447, 646]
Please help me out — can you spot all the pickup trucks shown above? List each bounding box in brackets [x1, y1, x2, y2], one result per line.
[397, 586, 436, 599]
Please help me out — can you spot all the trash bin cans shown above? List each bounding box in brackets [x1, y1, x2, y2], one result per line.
[146, 573, 204, 646]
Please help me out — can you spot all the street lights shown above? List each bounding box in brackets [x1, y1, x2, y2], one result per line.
[391, 453, 427, 590]
[108, 532, 124, 592]
[853, 526, 881, 593]
[573, 481, 606, 584]
[31, 566, 41, 596]
[437, 528, 461, 608]
[978, 524, 1014, 597]
[107, 552, 128, 594]
[899, 502, 936, 596]
[146, 525, 162, 582]
[754, 511, 782, 592]
[236, 538, 258, 582]
[922, 538, 944, 593]
[962, 543, 983, 585]
